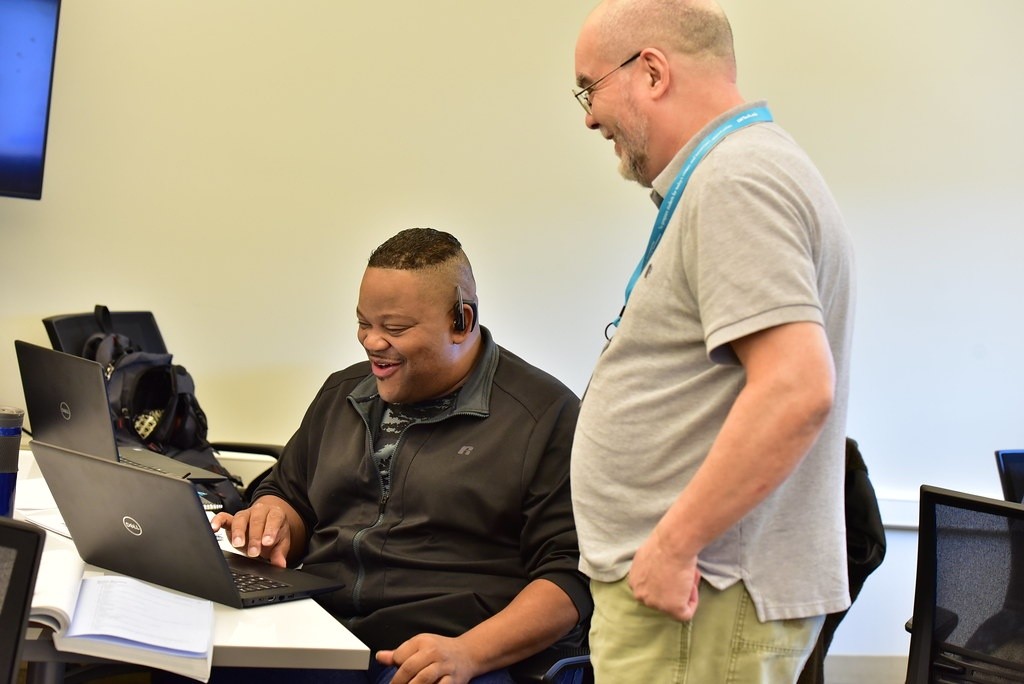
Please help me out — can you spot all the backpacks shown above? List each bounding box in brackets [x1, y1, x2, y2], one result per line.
[82, 305, 211, 456]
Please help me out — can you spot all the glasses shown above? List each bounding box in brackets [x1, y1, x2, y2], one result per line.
[576, 51, 642, 112]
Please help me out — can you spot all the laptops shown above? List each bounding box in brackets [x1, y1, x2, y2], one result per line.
[28, 441, 347, 610]
[14, 340, 228, 484]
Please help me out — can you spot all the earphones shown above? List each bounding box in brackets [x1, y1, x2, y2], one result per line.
[452, 284, 466, 331]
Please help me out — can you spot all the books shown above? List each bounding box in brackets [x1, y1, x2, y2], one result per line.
[30, 550, 215, 683]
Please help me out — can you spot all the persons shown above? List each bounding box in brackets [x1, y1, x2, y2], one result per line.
[570, 0, 856, 684]
[209, 228, 595, 684]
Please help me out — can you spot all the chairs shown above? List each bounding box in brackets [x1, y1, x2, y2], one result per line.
[40, 311, 287, 497]
[905, 484, 1024, 684]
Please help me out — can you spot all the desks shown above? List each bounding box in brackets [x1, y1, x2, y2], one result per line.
[0, 449, 372, 684]
